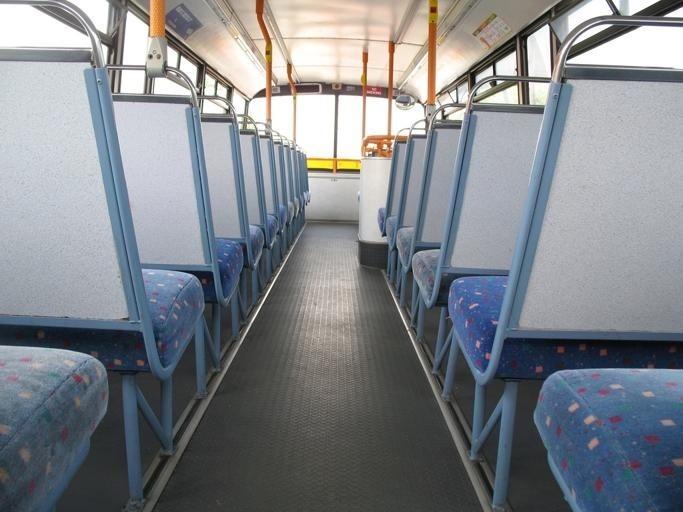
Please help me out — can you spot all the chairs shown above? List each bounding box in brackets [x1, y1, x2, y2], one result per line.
[0, 0, 212, 512]
[104, 52, 311, 373]
[1, 340, 113, 511]
[529, 364, 683, 511]
[375, 69, 551, 469]
[445, 15, 683, 512]
